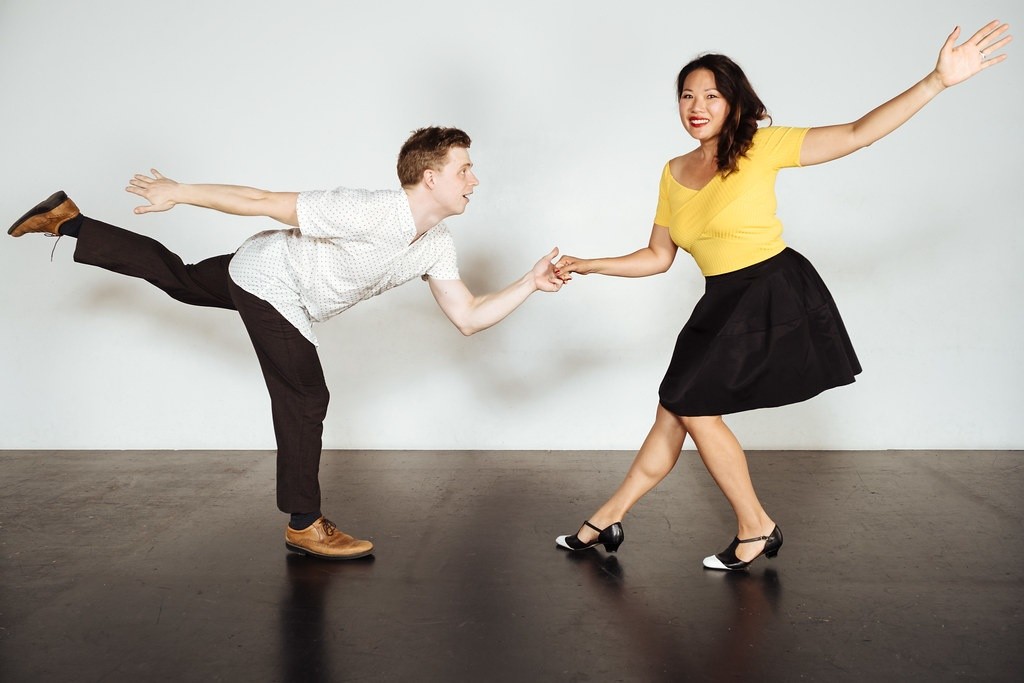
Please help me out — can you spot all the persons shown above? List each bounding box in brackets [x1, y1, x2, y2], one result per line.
[553, 19, 1014, 571]
[7, 126, 573, 561]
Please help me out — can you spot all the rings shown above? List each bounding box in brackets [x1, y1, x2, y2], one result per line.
[979, 51, 986, 58]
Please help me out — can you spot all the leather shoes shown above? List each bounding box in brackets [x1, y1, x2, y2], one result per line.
[8, 191, 80, 261]
[285, 514, 376, 560]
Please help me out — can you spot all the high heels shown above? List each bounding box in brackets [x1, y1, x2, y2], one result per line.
[556, 520, 624, 553]
[703, 524, 783, 570]
